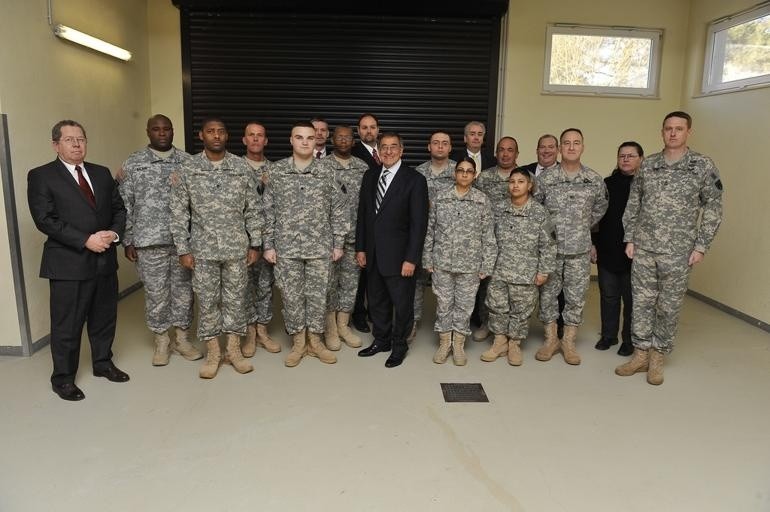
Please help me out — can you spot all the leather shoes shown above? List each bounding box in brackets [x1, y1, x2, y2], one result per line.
[596, 336, 618, 350]
[52, 381, 85, 401]
[352, 318, 370, 333]
[358, 340, 391, 356]
[385, 347, 409, 367]
[94, 363, 129, 382]
[618, 342, 634, 356]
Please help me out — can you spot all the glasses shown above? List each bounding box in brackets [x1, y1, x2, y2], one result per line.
[619, 153, 637, 161]
[456, 168, 474, 175]
[59, 137, 86, 144]
[334, 135, 353, 142]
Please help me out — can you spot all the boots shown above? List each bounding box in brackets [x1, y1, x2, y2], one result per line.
[647, 348, 664, 385]
[152, 311, 363, 380]
[433, 328, 583, 366]
[615, 348, 649, 376]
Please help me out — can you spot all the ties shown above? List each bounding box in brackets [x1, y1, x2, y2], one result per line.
[75, 165, 96, 210]
[373, 149, 382, 166]
[317, 152, 321, 159]
[375, 170, 391, 215]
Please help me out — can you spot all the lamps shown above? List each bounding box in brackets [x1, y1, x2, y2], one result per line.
[54, 20, 133, 62]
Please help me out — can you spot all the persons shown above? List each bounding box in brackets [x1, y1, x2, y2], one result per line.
[531, 128, 609, 365]
[407, 129, 457, 345]
[480, 168, 559, 365]
[615, 111, 725, 385]
[449, 120, 498, 178]
[27, 120, 129, 400]
[262, 120, 345, 368]
[590, 141, 644, 356]
[351, 114, 383, 333]
[422, 157, 498, 365]
[241, 118, 281, 358]
[310, 116, 331, 158]
[472, 136, 546, 342]
[521, 134, 563, 341]
[320, 124, 370, 351]
[354, 131, 429, 368]
[115, 114, 203, 366]
[168, 115, 267, 379]
